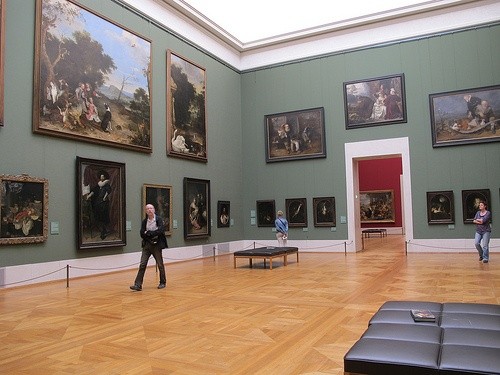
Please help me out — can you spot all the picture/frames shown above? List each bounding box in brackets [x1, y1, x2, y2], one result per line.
[312, 196, 336, 228]
[182, 176, 212, 242]
[461, 189, 491, 225]
[427, 84, 500, 150]
[284, 197, 308, 227]
[0, 172, 49, 245]
[141, 183, 174, 238]
[165, 47, 209, 164]
[256, 199, 276, 228]
[31, 0, 155, 155]
[425, 190, 456, 226]
[342, 71, 407, 132]
[216, 200, 231, 229]
[74, 155, 128, 252]
[263, 105, 327, 164]
[359, 188, 396, 224]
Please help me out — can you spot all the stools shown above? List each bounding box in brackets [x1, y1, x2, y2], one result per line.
[362, 228, 387, 238]
[341, 297, 500, 375]
[234, 246, 300, 271]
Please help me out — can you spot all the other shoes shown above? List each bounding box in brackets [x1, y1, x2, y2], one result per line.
[158, 284, 166, 288]
[479, 257, 482, 261]
[130, 285, 142, 291]
[483, 260, 488, 263]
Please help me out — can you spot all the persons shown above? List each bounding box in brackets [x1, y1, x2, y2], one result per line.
[275, 210, 288, 247]
[128, 204, 168, 291]
[473, 200, 491, 263]
